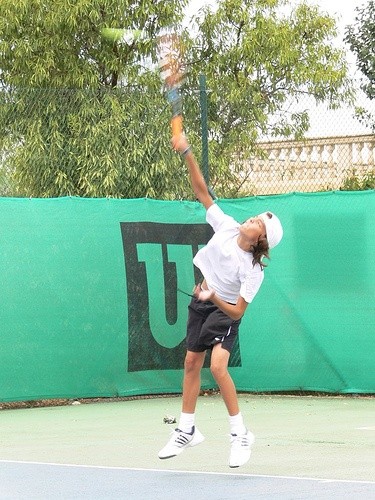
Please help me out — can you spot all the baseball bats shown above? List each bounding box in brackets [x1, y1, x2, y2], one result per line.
[156, 40, 186, 147]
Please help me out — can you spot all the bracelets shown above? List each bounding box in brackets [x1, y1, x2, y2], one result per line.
[180, 145, 191, 156]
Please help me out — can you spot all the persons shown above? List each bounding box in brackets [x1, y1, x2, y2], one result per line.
[157, 131, 284, 468]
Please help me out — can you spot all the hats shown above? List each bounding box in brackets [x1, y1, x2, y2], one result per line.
[258, 212, 284, 249]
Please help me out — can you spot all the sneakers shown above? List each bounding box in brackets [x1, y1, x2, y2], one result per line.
[158, 424, 205, 460]
[228, 426, 254, 469]
